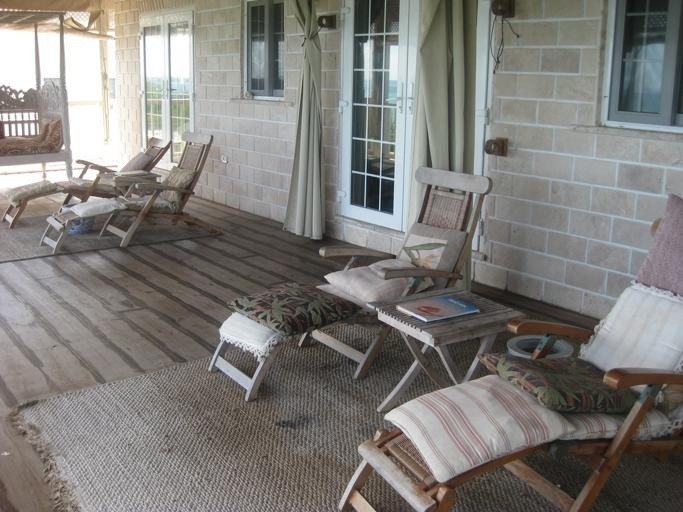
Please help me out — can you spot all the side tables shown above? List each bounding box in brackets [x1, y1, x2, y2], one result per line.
[365, 284, 528, 414]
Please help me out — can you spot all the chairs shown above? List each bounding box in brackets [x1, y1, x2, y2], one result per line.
[0, 132, 226, 254]
[206, 163, 495, 404]
[338, 193, 683, 510]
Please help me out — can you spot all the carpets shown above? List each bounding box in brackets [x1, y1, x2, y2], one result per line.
[0, 210, 226, 264]
[8, 285, 683, 512]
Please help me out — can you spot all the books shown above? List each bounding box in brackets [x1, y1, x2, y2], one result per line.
[396, 295, 480, 324]
[114, 170, 148, 177]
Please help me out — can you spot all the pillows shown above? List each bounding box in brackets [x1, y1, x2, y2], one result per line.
[323, 221, 436, 308]
[226, 281, 362, 339]
[481, 352, 659, 418]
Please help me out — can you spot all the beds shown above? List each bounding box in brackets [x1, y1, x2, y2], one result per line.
[0, 80, 74, 180]
